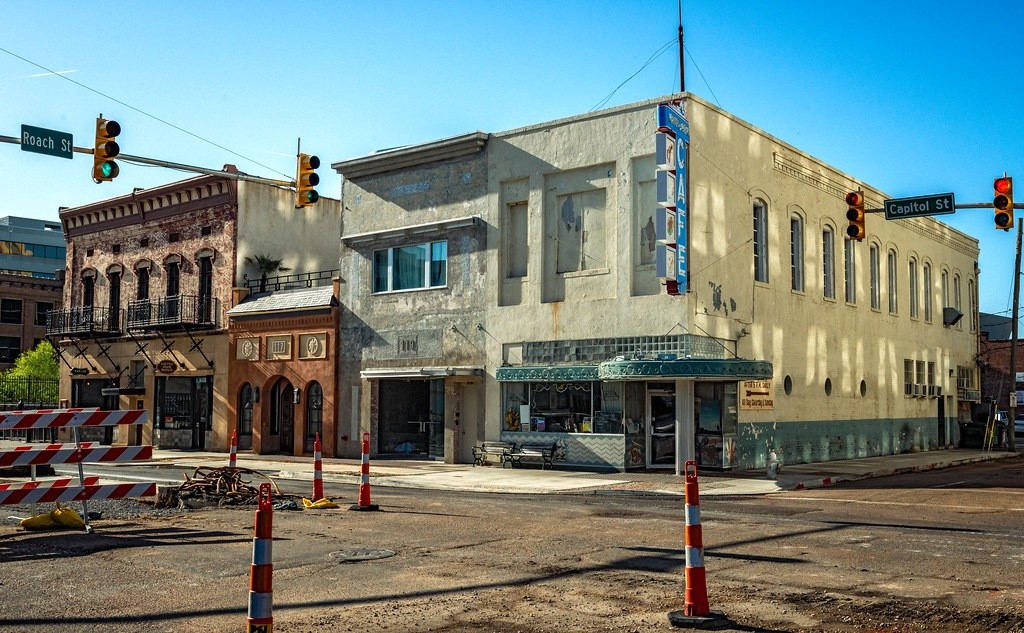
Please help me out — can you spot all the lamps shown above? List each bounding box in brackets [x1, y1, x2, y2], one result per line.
[292, 387, 300, 404]
[253, 385, 260, 403]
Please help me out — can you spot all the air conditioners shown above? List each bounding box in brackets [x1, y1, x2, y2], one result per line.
[920, 384, 927, 398]
[929, 384, 942, 398]
[905, 382, 920, 397]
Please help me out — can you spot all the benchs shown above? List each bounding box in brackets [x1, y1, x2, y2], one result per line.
[510, 443, 557, 470]
[471, 440, 517, 469]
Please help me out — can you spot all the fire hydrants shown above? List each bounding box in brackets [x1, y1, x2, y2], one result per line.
[766, 449, 781, 480]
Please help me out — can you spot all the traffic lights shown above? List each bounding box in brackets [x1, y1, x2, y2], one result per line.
[90, 112, 121, 185]
[846, 191, 865, 241]
[295, 152, 319, 210]
[993, 176, 1014, 232]
